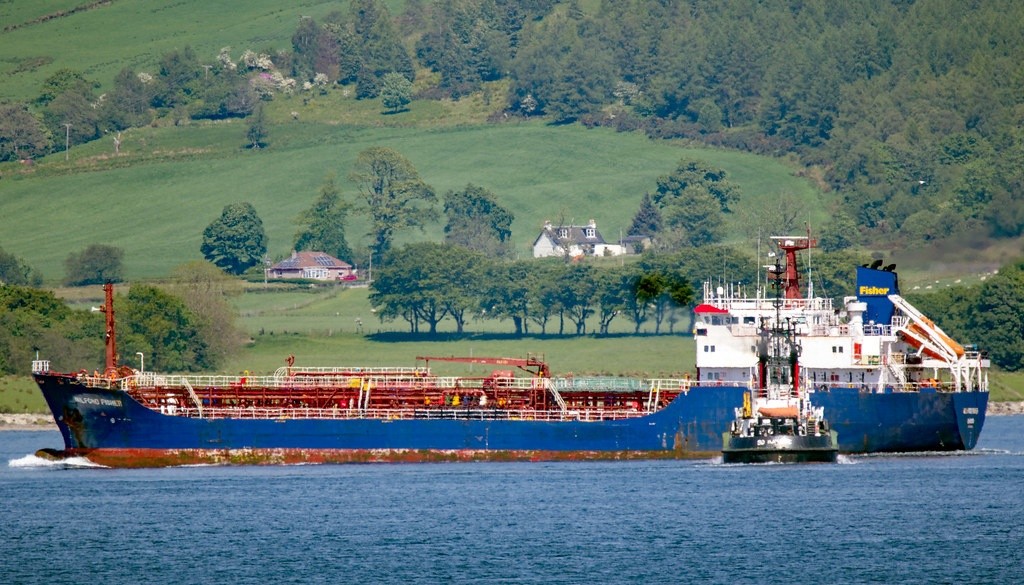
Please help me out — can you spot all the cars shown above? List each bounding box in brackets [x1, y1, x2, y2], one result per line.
[341, 275, 357, 280]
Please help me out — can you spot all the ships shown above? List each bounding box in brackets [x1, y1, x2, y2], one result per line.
[32, 234, 991, 469]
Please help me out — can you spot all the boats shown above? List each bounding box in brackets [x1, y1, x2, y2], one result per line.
[721, 259, 840, 464]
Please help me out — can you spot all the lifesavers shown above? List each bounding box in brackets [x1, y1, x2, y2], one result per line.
[287, 405, 293, 413]
[930, 379, 936, 387]
[79, 369, 88, 377]
